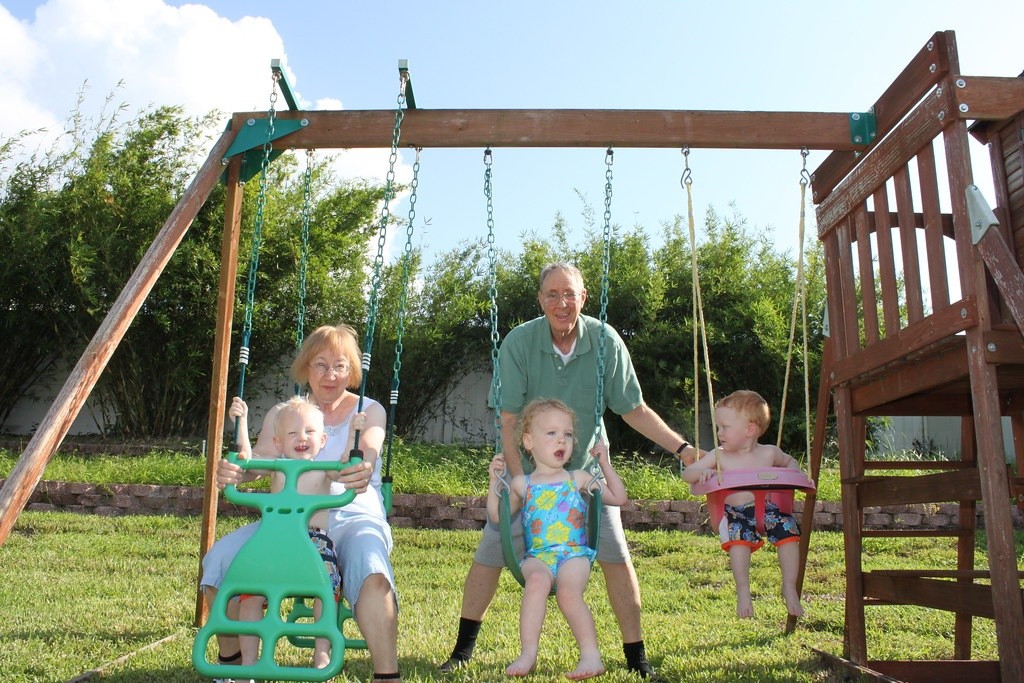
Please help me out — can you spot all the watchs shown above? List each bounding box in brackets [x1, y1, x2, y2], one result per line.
[673, 442, 690, 460]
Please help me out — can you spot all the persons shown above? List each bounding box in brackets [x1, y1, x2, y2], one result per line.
[200, 324, 402, 683]
[485, 398, 627, 681]
[683, 389, 805, 619]
[229, 396, 367, 683]
[437, 262, 709, 683]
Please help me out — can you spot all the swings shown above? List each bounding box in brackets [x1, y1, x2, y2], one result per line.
[188, 70, 423, 681]
[482, 153, 615, 597]
[679, 165, 821, 539]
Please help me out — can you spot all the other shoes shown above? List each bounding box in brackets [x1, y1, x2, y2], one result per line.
[211, 655, 264, 683]
[437, 657, 467, 674]
[632, 668, 669, 683]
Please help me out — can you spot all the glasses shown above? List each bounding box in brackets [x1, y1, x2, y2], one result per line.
[539, 291, 582, 305]
[306, 360, 350, 376]
